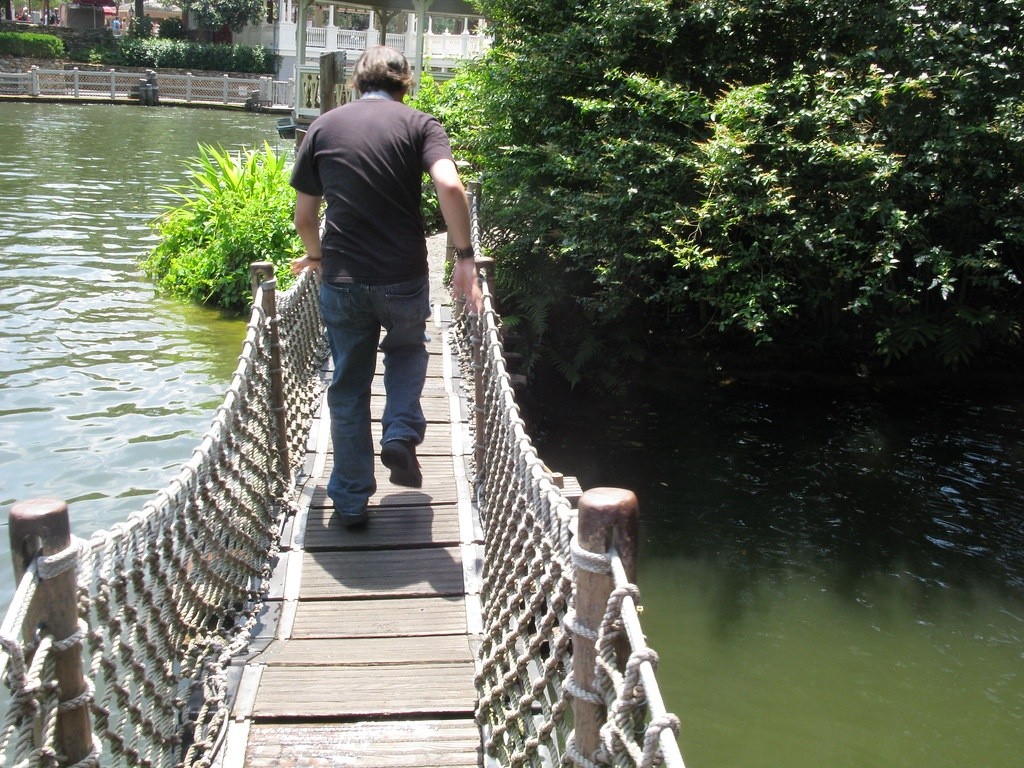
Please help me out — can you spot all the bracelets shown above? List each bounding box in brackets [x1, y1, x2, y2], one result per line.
[308, 255, 323, 262]
[456, 243, 474, 260]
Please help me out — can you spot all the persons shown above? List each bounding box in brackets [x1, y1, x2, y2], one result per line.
[150, 21, 160, 35]
[290, 46, 484, 526]
[113, 16, 127, 31]
[40, 7, 60, 26]
[16, 6, 32, 22]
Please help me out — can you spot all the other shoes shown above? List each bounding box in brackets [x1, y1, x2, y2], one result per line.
[333, 502, 368, 526]
[381, 439, 422, 487]
